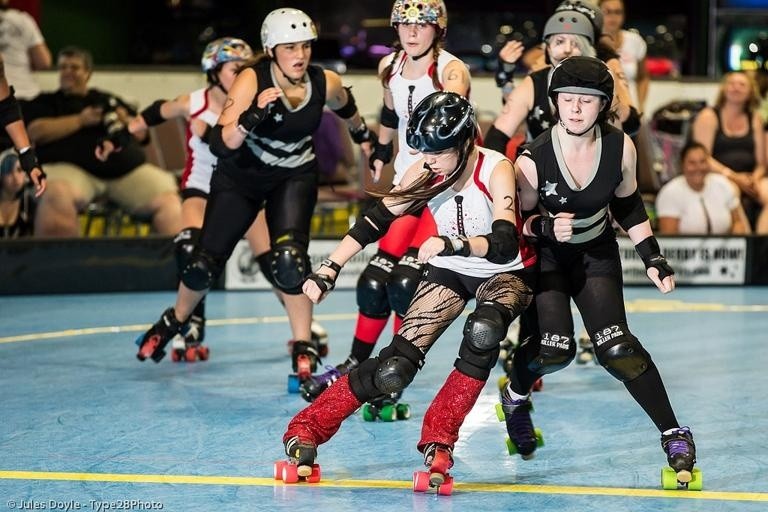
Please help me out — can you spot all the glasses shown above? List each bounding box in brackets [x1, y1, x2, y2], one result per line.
[604, 6, 624, 16]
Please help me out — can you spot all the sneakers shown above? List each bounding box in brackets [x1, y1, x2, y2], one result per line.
[412, 441, 455, 498]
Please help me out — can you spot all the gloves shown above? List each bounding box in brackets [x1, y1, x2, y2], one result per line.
[95, 123, 134, 152]
[347, 117, 373, 144]
[17, 147, 47, 182]
[307, 257, 342, 294]
[236, 93, 268, 135]
[634, 235, 675, 278]
[368, 140, 395, 171]
[431, 234, 470, 260]
[528, 216, 558, 244]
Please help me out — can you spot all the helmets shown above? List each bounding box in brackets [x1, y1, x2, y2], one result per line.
[542, 10, 596, 43]
[557, 1, 605, 34]
[389, 0, 447, 32]
[200, 38, 254, 71]
[404, 93, 477, 154]
[260, 7, 318, 49]
[547, 55, 614, 101]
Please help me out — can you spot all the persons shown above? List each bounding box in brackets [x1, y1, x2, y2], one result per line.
[693, 73, 768, 235]
[284, 93, 531, 469]
[0, 57, 46, 200]
[1, 1, 50, 98]
[97, 37, 328, 348]
[0, 151, 36, 240]
[133, 9, 381, 376]
[656, 141, 749, 237]
[599, 0, 659, 193]
[27, 45, 186, 240]
[502, 55, 697, 468]
[310, 0, 470, 397]
[484, 11, 638, 376]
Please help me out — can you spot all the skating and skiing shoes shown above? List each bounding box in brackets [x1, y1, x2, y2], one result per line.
[311, 323, 329, 358]
[658, 430, 703, 490]
[169, 316, 212, 365]
[286, 342, 320, 395]
[495, 382, 545, 461]
[270, 431, 323, 485]
[497, 341, 544, 396]
[298, 357, 361, 401]
[136, 309, 180, 365]
[363, 389, 410, 424]
[578, 335, 598, 366]
